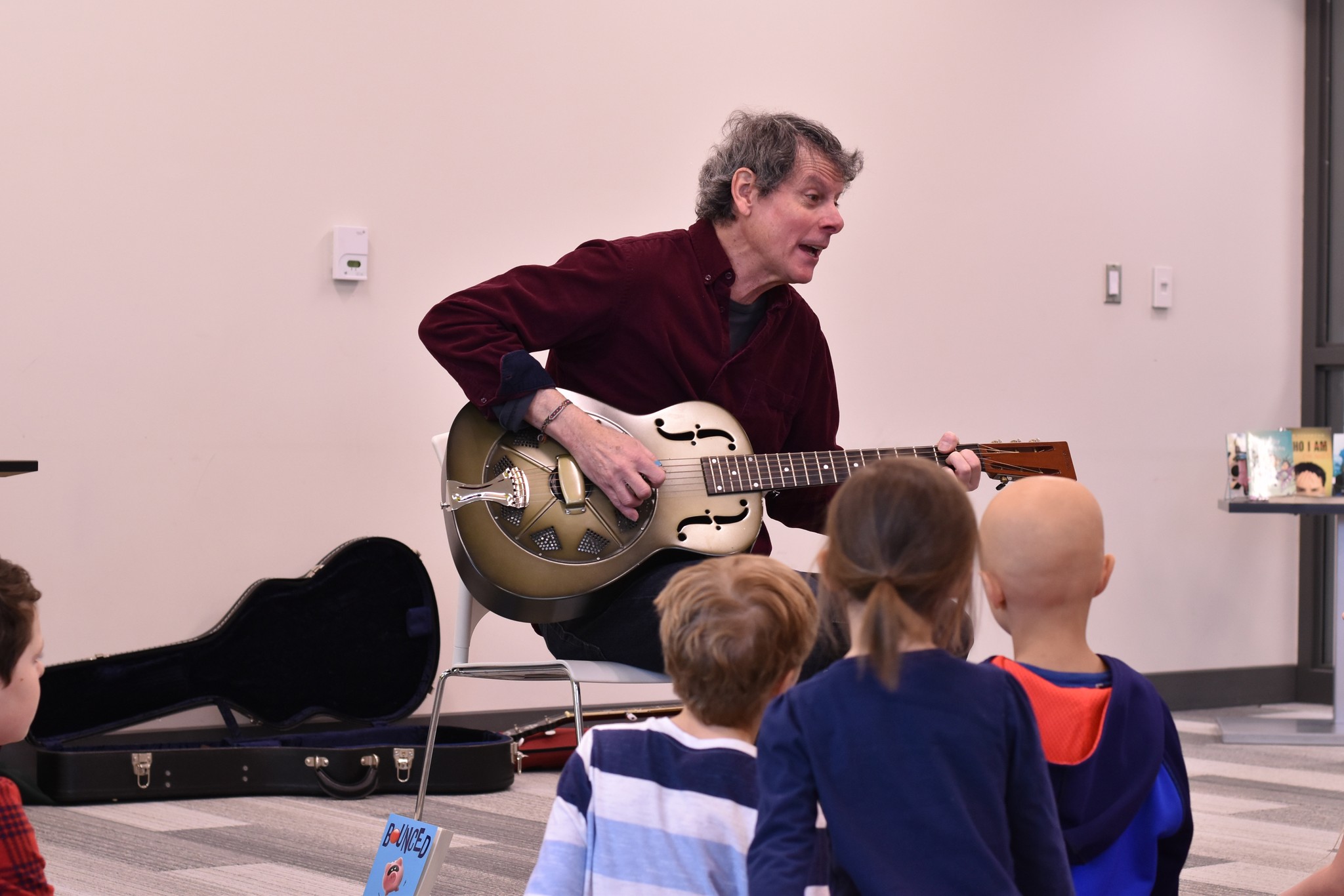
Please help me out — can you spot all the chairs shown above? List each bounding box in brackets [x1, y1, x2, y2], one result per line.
[414, 429, 673, 821]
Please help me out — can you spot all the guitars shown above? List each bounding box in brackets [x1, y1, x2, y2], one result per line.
[438, 379, 1080, 626]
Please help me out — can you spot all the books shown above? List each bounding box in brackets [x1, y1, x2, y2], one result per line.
[361, 812, 455, 896]
[1225, 426, 1344, 501]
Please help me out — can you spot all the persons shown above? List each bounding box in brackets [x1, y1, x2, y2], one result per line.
[978, 476, 1196, 896]
[418, 111, 981, 687]
[0, 556, 68, 896]
[519, 554, 818, 896]
[1278, 835, 1344, 896]
[750, 451, 1072, 896]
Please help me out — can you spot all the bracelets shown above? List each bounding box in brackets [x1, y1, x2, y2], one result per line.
[538, 398, 573, 444]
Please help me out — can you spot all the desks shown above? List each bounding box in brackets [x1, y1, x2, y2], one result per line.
[1217, 494, 1344, 746]
